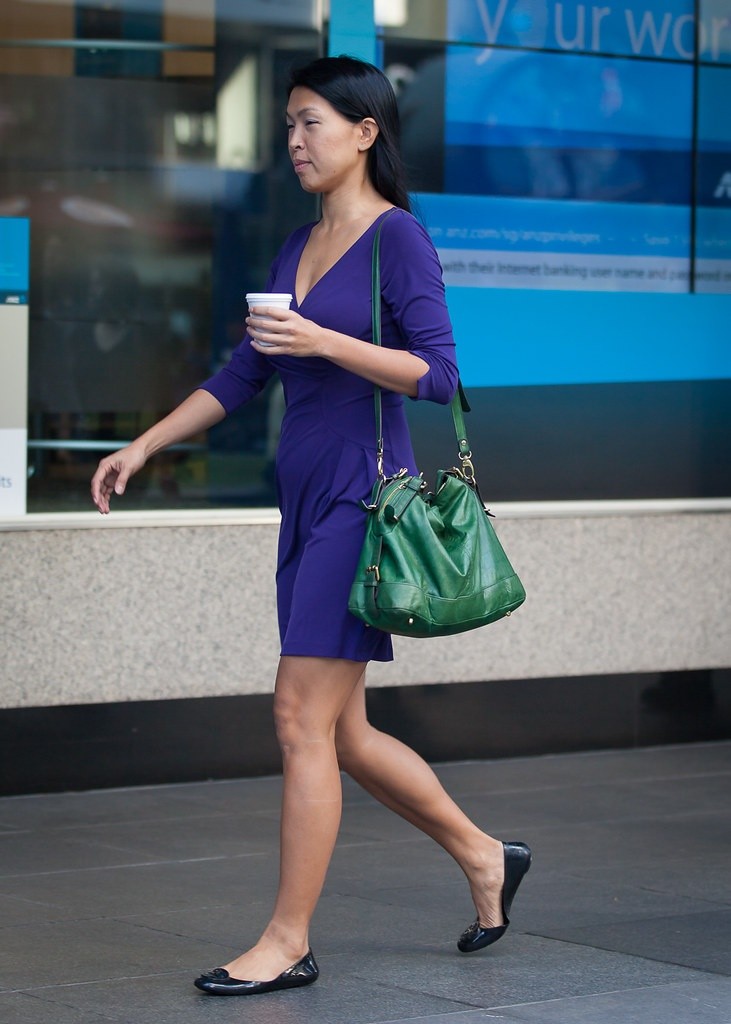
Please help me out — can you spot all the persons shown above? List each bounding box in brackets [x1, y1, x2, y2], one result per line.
[90, 56, 532, 993]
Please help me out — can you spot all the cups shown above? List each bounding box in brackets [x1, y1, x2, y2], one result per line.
[246, 293, 293, 347]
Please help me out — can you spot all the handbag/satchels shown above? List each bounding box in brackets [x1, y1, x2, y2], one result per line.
[346, 465, 526, 637]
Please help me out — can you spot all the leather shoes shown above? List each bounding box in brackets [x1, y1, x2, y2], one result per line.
[194, 947, 317, 995]
[457, 839, 530, 951]
